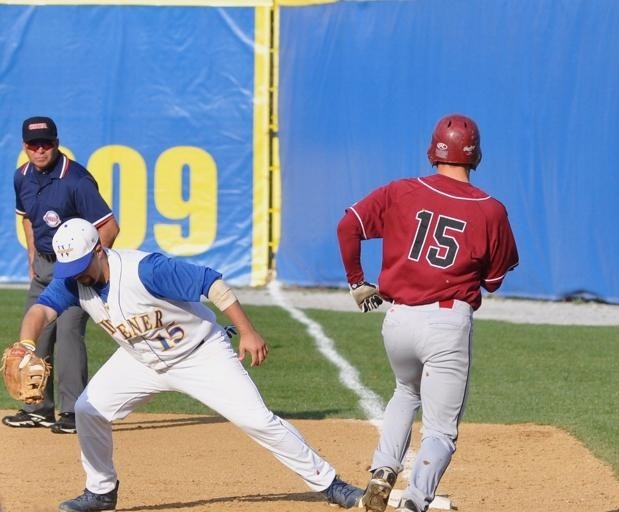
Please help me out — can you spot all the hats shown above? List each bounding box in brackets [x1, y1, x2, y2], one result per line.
[51, 218, 99, 279]
[22, 116, 57, 141]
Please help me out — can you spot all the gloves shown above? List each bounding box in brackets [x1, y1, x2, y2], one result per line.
[348, 281, 383, 313]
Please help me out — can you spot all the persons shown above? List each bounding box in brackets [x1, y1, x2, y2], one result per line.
[0, 115, 120, 436]
[335, 113, 521, 511]
[0, 217, 373, 511]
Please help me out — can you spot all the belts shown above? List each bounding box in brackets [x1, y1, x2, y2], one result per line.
[40, 253, 57, 263]
[394, 299, 454, 309]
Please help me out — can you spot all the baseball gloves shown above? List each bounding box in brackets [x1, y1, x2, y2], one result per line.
[0, 342, 52, 406]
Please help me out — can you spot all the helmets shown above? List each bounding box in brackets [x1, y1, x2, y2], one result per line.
[427, 115, 483, 171]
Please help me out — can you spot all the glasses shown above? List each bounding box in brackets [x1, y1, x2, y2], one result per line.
[25, 141, 54, 152]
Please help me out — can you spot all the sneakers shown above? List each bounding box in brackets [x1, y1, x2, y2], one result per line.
[363, 466, 397, 512]
[51, 413, 76, 433]
[58, 480, 119, 512]
[396, 494, 421, 512]
[2, 407, 56, 428]
[320, 474, 364, 510]
